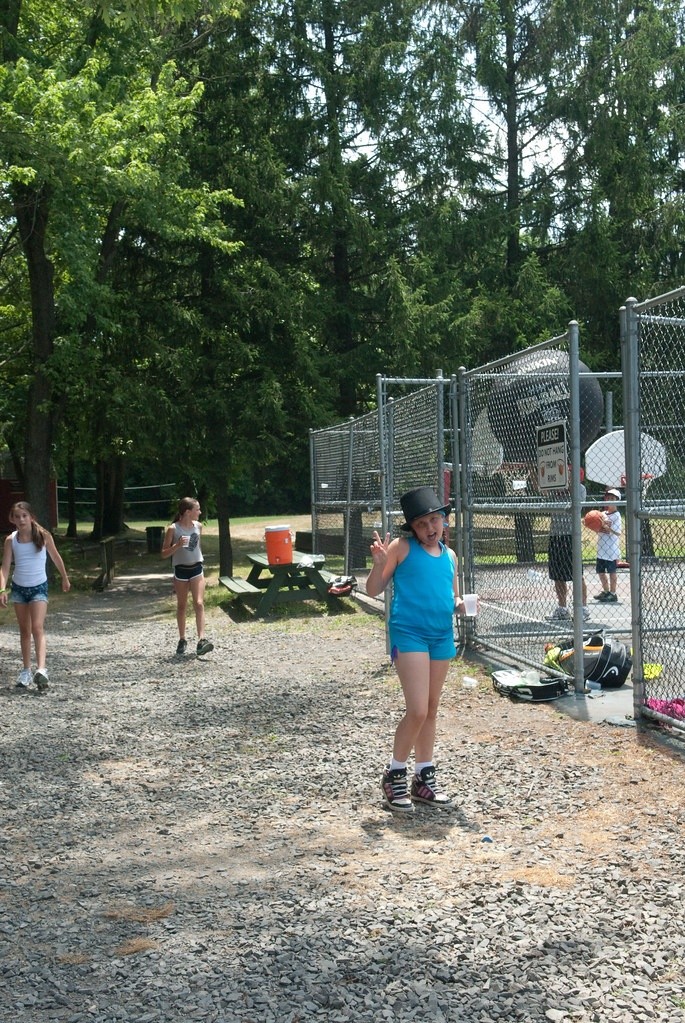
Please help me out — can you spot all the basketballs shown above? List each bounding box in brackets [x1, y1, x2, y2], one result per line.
[584, 510, 603, 530]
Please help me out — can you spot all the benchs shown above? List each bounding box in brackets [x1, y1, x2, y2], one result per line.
[218, 570, 352, 605]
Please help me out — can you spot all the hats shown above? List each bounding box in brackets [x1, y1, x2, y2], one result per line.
[567, 465, 585, 481]
[605, 489, 621, 501]
[399, 486, 452, 532]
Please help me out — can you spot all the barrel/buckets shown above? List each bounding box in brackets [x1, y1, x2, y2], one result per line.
[263, 525, 292, 565]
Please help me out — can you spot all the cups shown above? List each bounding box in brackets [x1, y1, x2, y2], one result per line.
[585, 679, 601, 690]
[182, 536, 191, 547]
[462, 594, 478, 616]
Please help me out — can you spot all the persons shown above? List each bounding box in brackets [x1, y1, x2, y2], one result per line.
[0, 501, 71, 689]
[583, 488, 623, 601]
[544, 465, 591, 621]
[365, 486, 479, 811]
[161, 497, 214, 655]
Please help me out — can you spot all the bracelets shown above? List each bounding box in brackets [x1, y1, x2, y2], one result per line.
[0, 589, 6, 593]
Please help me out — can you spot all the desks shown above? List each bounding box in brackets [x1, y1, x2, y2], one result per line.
[246, 550, 342, 619]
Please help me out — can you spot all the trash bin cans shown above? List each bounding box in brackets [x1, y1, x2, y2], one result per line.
[145, 526, 164, 552]
[265, 524, 293, 566]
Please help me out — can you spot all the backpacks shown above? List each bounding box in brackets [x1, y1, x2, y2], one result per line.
[492, 665, 569, 701]
[328, 576, 357, 597]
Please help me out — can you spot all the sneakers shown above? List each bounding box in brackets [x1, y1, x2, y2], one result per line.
[380, 764, 414, 812]
[545, 607, 571, 620]
[16, 670, 32, 687]
[594, 591, 617, 602]
[33, 670, 49, 691]
[411, 766, 453, 808]
[177, 640, 187, 653]
[197, 638, 214, 655]
[571, 606, 591, 621]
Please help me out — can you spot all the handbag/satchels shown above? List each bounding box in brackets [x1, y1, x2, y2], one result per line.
[543, 630, 633, 688]
